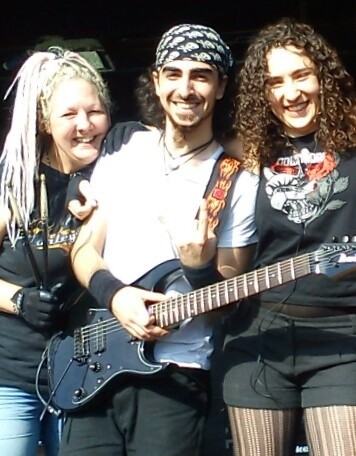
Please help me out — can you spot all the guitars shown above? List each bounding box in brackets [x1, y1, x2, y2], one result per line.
[42, 233, 355, 411]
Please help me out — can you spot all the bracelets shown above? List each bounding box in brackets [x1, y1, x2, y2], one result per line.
[180, 255, 228, 290]
[88, 269, 130, 316]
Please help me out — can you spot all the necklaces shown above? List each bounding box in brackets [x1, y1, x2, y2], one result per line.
[160, 131, 215, 177]
[45, 147, 51, 166]
[163, 130, 213, 170]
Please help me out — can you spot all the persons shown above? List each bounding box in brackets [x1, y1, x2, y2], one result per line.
[215, 24, 356, 456]
[56, 21, 261, 456]
[0, 44, 117, 456]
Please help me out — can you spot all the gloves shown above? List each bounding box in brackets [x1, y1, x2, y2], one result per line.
[12, 283, 63, 328]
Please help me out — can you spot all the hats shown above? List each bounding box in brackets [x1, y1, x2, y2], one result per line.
[154, 24, 233, 76]
